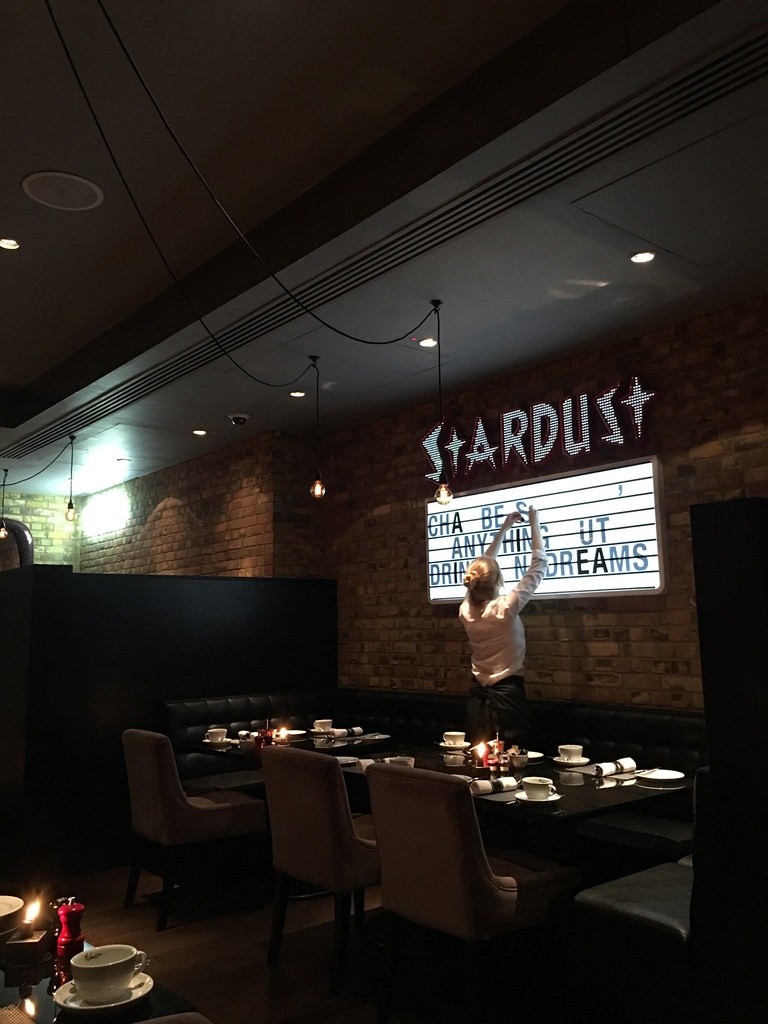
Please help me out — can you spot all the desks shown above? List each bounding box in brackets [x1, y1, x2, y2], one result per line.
[0, 887, 198, 1024]
[333, 747, 696, 998]
[191, 733, 405, 901]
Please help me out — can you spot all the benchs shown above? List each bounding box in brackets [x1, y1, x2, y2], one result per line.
[162, 687, 710, 1024]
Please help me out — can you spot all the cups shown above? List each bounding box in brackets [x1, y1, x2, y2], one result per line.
[71, 945, 148, 1002]
[205, 729, 227, 741]
[239, 740, 255, 750]
[314, 719, 332, 731]
[558, 745, 583, 761]
[522, 777, 556, 799]
[389, 757, 414, 769]
[443, 731, 465, 744]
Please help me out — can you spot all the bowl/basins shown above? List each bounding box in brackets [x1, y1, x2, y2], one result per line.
[0, 895, 25, 934]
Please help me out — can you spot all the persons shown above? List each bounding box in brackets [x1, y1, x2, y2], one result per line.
[456, 504, 548, 749]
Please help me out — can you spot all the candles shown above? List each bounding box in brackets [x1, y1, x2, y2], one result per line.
[20, 896, 43, 940]
[470, 737, 491, 766]
[486, 730, 505, 754]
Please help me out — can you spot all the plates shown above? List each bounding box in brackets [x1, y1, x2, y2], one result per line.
[439, 742, 471, 749]
[276, 729, 306, 734]
[310, 729, 336, 733]
[356, 734, 390, 738]
[634, 769, 684, 780]
[54, 972, 153, 1009]
[0, 922, 21, 936]
[133, 1012, 211, 1024]
[335, 757, 359, 763]
[503, 751, 543, 758]
[553, 757, 590, 765]
[514, 792, 560, 801]
[203, 738, 231, 744]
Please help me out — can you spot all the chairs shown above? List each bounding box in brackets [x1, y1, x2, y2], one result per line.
[121, 727, 267, 931]
[366, 763, 571, 1024]
[260, 744, 382, 1002]
